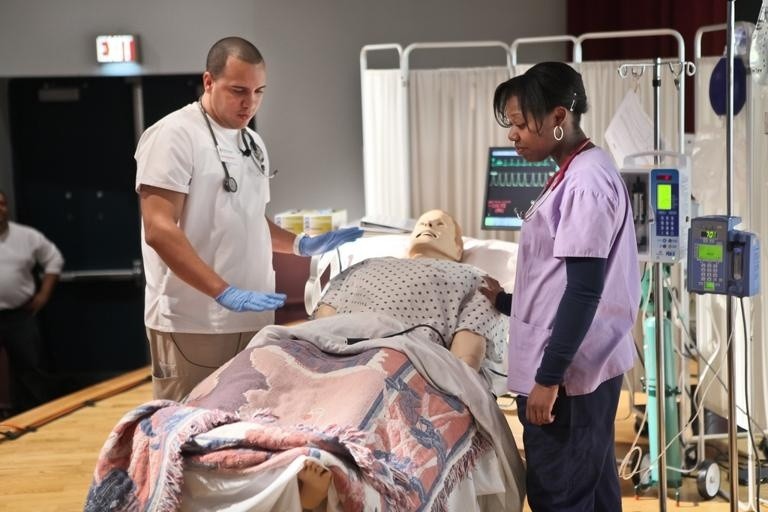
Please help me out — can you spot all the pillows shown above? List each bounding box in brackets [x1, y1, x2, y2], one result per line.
[350, 233, 519, 294]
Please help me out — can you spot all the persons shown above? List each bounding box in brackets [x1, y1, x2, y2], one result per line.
[295, 207, 507, 512]
[134, 36, 364, 404]
[476, 61, 643, 511]
[0, 190, 65, 411]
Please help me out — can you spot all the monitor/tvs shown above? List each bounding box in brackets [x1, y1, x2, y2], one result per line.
[481, 148, 563, 231]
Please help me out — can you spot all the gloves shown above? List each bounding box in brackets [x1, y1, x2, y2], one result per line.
[299, 227, 363, 257]
[215, 285, 287, 312]
